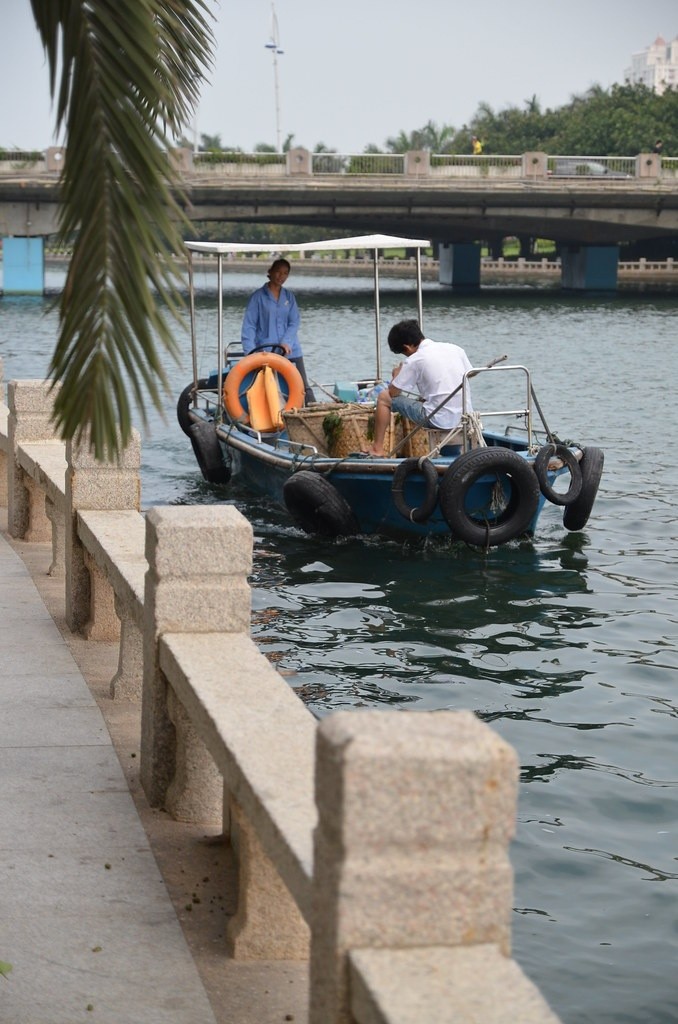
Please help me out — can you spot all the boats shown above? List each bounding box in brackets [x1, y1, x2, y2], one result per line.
[175, 230, 605, 549]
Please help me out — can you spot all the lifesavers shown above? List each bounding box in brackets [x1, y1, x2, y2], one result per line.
[391, 457, 439, 523]
[441, 444, 541, 546]
[187, 422, 230, 486]
[222, 352, 305, 433]
[281, 470, 359, 544]
[534, 443, 582, 505]
[561, 445, 604, 531]
[177, 377, 211, 438]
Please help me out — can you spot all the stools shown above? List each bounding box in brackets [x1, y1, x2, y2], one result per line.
[426, 427, 468, 456]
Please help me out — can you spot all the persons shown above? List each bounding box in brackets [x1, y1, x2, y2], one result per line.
[472, 137, 482, 155]
[482, 137, 491, 155]
[653, 140, 663, 155]
[475, 236, 556, 261]
[242, 258, 316, 408]
[364, 319, 472, 456]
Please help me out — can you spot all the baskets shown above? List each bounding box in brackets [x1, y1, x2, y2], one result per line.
[280, 402, 441, 458]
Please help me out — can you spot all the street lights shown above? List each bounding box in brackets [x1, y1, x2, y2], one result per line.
[263, 36, 286, 152]
